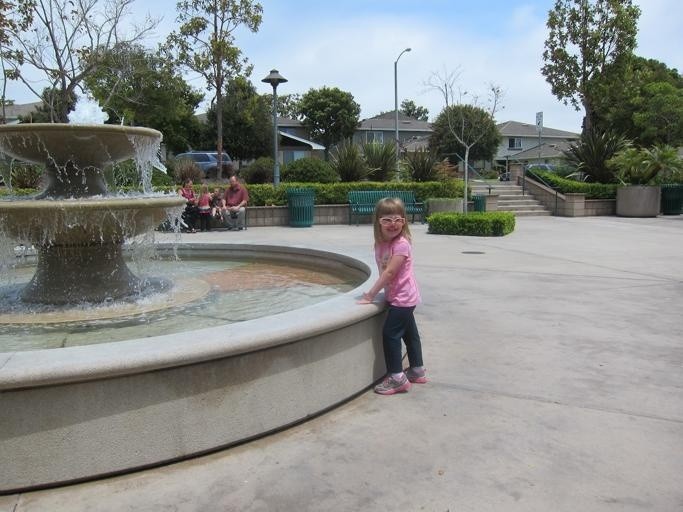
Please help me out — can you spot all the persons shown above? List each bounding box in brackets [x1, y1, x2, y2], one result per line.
[210, 188, 224, 221]
[177, 175, 198, 233]
[354, 197, 429, 395]
[196, 184, 213, 232]
[222, 176, 249, 231]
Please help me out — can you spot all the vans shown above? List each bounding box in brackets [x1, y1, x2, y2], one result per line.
[173, 151, 234, 180]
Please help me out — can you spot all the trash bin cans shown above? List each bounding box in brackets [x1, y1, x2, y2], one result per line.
[286, 188, 314, 227]
[473, 196, 484, 211]
[661, 184, 683, 215]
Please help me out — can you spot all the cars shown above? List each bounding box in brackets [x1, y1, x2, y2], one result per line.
[497, 172, 509, 181]
[524, 163, 556, 171]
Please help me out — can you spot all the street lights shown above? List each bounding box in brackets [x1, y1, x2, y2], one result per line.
[392, 46, 412, 180]
[261, 68, 289, 185]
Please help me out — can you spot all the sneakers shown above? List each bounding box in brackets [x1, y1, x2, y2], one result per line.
[374, 373, 410, 394]
[405, 368, 426, 383]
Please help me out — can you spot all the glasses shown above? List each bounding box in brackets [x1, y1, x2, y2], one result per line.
[378, 218, 405, 226]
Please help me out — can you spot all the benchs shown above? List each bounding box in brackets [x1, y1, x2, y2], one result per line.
[347, 190, 428, 227]
[154, 188, 248, 231]
